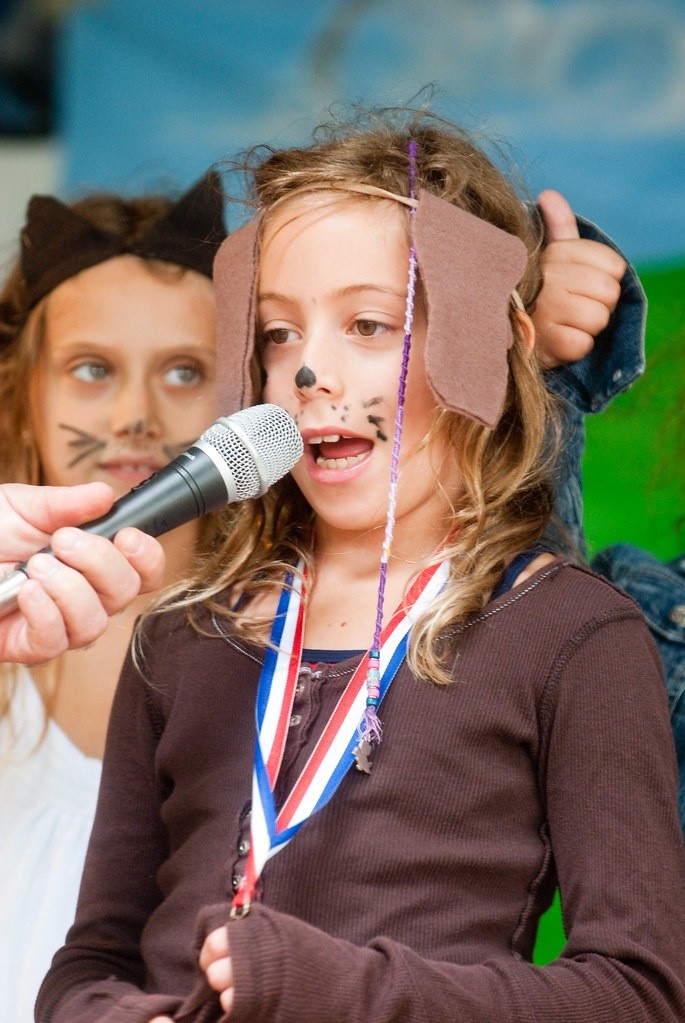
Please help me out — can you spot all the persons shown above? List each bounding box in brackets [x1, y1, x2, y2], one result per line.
[0, 480, 165, 664]
[531, 189, 685, 832]
[34, 126, 685, 1023]
[0, 170, 228, 1023]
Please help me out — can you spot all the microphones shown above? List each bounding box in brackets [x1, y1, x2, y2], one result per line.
[0, 404, 304, 623]
